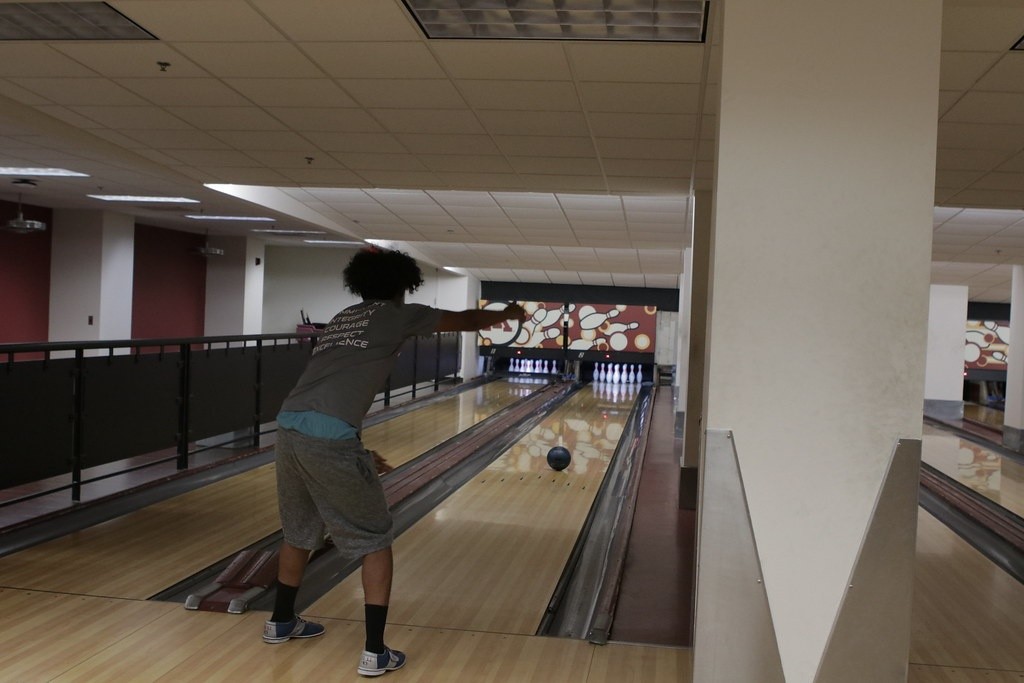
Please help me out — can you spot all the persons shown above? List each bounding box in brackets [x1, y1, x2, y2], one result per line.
[263, 247, 526, 676]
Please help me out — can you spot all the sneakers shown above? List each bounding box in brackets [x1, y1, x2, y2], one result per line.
[356, 643, 406, 675]
[262, 614, 326, 644]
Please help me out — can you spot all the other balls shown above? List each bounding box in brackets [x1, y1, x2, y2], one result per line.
[548, 447, 573, 471]
